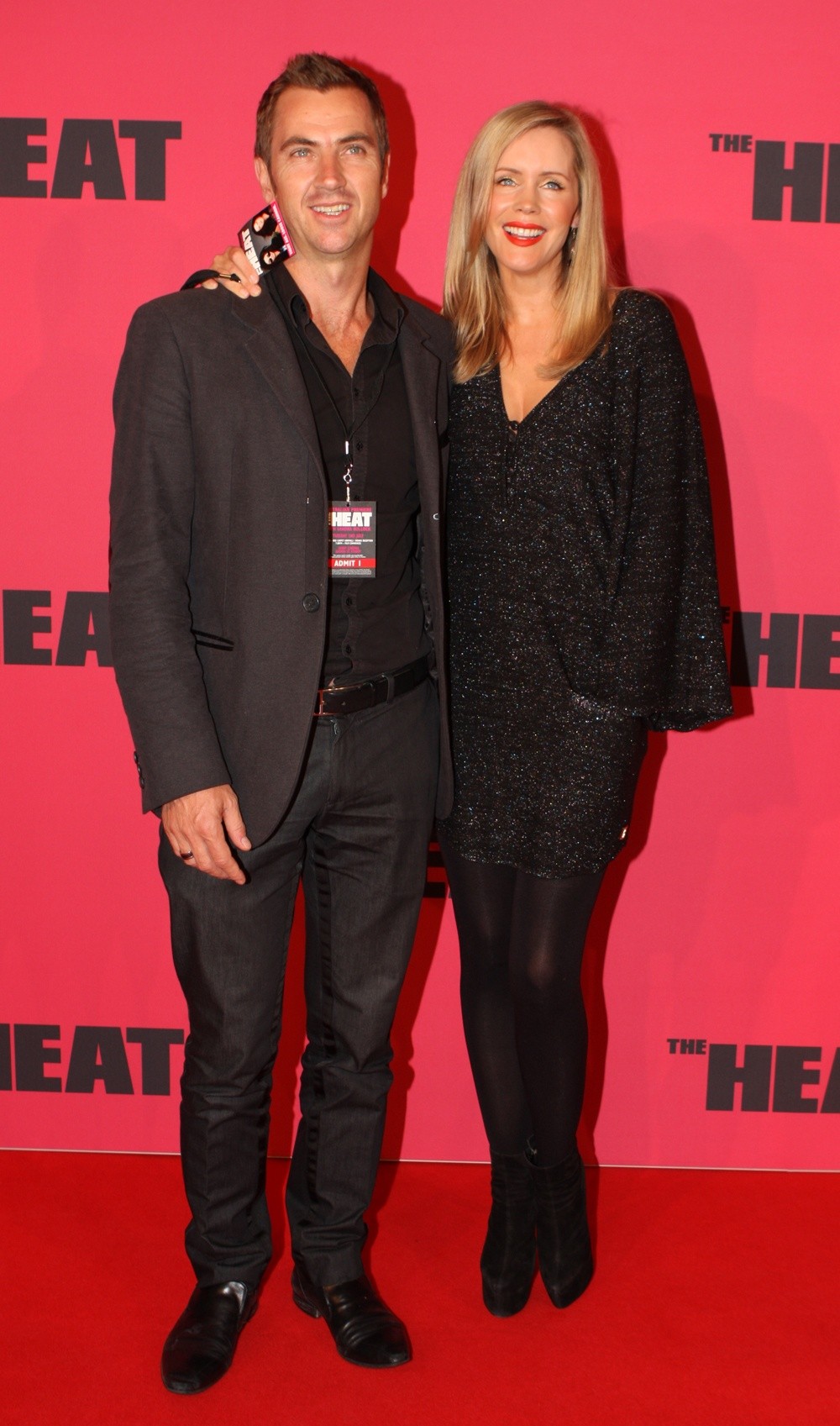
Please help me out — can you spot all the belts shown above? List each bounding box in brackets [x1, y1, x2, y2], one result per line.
[314, 652, 432, 718]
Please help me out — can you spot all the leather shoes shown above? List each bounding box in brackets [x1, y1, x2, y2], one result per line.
[160, 1273, 263, 1395]
[290, 1266, 418, 1368]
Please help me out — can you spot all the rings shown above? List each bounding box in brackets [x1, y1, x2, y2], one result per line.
[180, 851, 193, 859]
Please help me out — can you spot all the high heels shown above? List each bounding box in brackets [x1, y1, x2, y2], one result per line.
[478, 1183, 535, 1316]
[532, 1175, 597, 1308]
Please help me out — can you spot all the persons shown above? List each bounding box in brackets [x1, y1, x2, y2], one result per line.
[193, 100, 734, 1318]
[252, 208, 288, 269]
[107, 54, 457, 1394]
